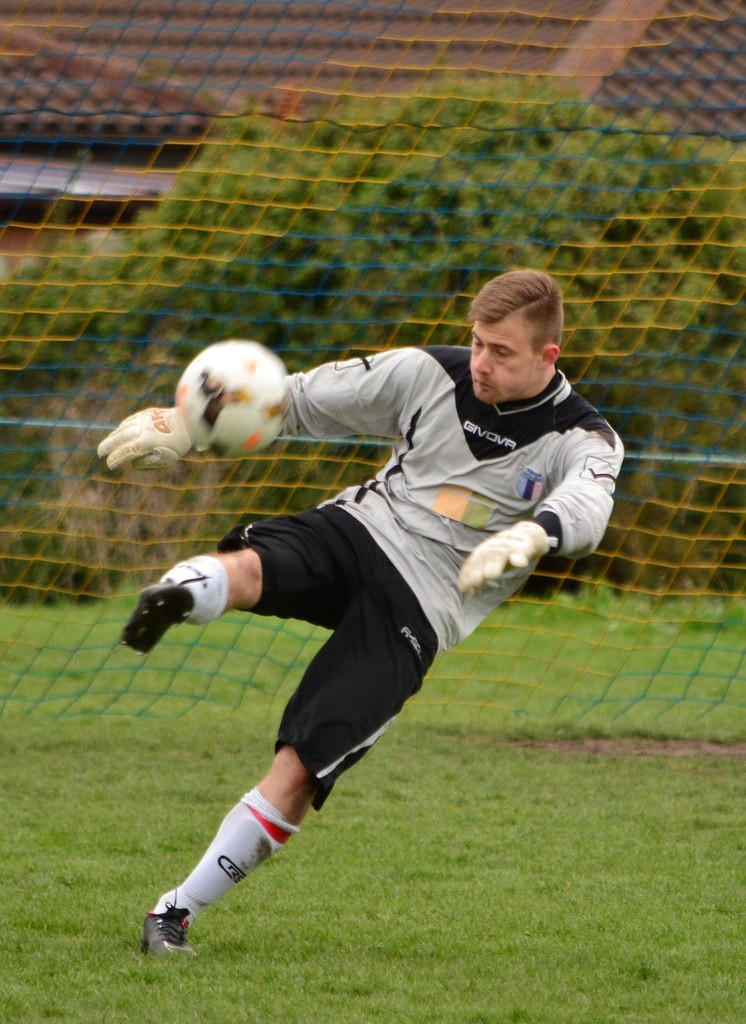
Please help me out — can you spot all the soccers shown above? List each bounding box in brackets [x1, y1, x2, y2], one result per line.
[176, 340, 289, 461]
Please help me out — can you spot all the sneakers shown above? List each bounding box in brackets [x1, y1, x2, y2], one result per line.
[140, 889, 198, 959]
[121, 577, 205, 654]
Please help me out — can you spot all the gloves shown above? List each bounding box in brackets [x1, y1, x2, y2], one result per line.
[459, 520, 549, 597]
[97, 408, 193, 470]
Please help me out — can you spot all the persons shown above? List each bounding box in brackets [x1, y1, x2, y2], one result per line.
[96, 271, 624, 954]
